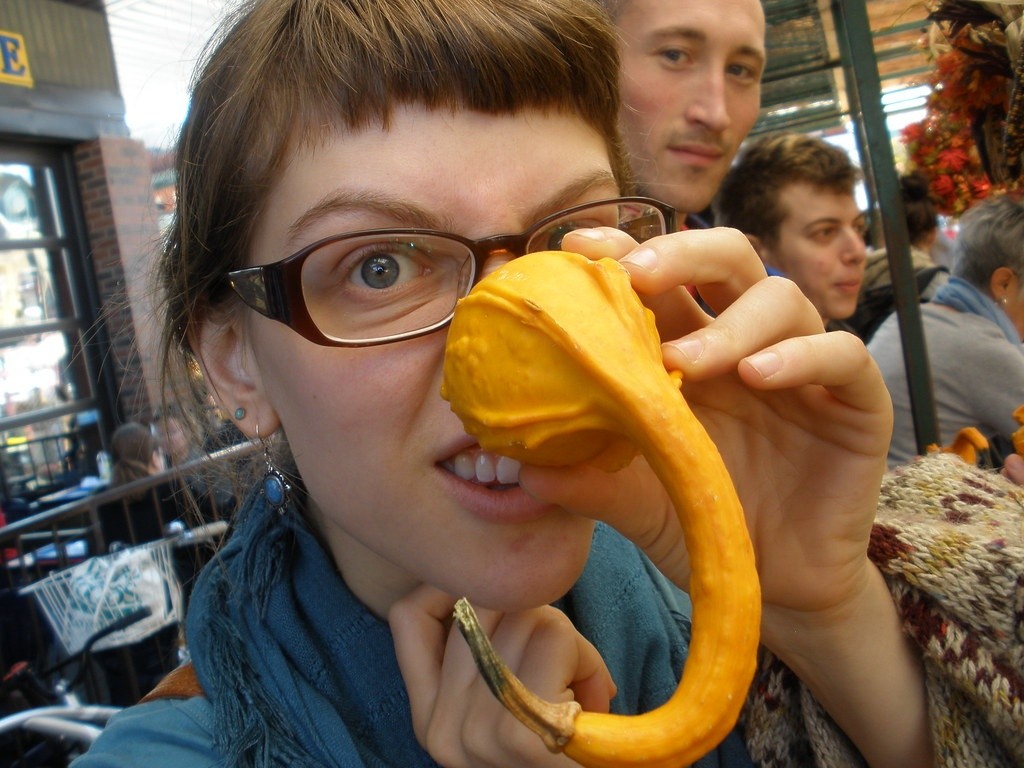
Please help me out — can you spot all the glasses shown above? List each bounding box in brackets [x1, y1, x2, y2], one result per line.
[215, 193, 677, 347]
[139, 419, 156, 436]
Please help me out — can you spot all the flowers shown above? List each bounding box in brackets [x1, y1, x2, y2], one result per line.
[897, 21, 1024, 226]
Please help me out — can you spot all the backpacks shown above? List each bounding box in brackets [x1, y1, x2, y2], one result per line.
[824, 264, 949, 346]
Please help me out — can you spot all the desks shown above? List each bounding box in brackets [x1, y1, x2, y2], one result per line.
[7, 539, 87, 568]
[35, 485, 93, 502]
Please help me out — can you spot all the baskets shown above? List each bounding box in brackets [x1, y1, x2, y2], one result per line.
[18, 531, 183, 656]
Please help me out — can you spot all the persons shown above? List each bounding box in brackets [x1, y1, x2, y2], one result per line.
[97, 400, 243, 702]
[64, 0, 930, 768]
[606, 0, 1023, 485]
